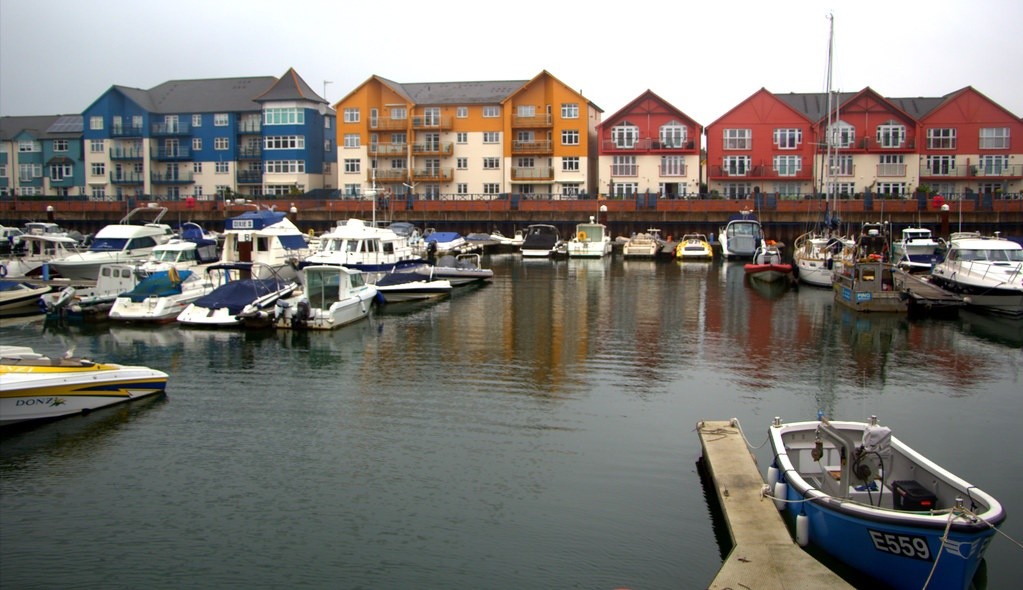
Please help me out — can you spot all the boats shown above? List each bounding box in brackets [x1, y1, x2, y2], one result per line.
[922, 236, 1023, 314]
[274, 263, 376, 331]
[623, 233, 661, 260]
[417, 253, 494, 286]
[675, 233, 714, 261]
[130, 164, 465, 281]
[832, 224, 907, 312]
[0, 222, 160, 318]
[568, 215, 612, 259]
[891, 210, 944, 271]
[176, 262, 297, 330]
[766, 414, 1006, 590]
[744, 240, 791, 284]
[365, 272, 451, 302]
[463, 232, 501, 245]
[0, 344, 170, 424]
[519, 225, 563, 257]
[717, 219, 765, 259]
[107, 269, 217, 320]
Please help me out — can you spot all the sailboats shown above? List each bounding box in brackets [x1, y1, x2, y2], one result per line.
[794, 13, 855, 287]
